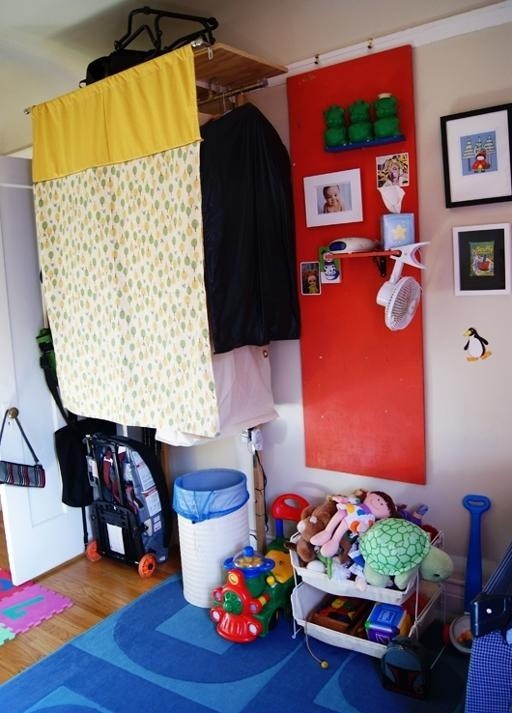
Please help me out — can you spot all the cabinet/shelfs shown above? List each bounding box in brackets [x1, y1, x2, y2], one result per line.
[288, 529, 450, 658]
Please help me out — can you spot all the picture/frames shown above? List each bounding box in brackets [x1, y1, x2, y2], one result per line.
[440, 101, 512, 208]
[451, 221, 512, 299]
[302, 168, 364, 229]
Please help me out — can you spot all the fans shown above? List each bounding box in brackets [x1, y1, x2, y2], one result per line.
[374, 239, 429, 333]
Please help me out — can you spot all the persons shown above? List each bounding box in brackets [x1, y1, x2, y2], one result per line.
[319, 184, 347, 212]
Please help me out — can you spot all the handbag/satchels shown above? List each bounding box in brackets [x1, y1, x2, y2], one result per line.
[53, 408, 120, 511]
[0, 407, 46, 488]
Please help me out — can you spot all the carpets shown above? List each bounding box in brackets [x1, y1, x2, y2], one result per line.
[0, 569, 457, 712]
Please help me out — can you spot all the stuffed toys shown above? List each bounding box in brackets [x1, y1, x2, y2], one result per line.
[298, 484, 454, 587]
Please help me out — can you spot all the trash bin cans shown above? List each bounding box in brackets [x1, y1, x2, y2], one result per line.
[173, 468, 249, 609]
